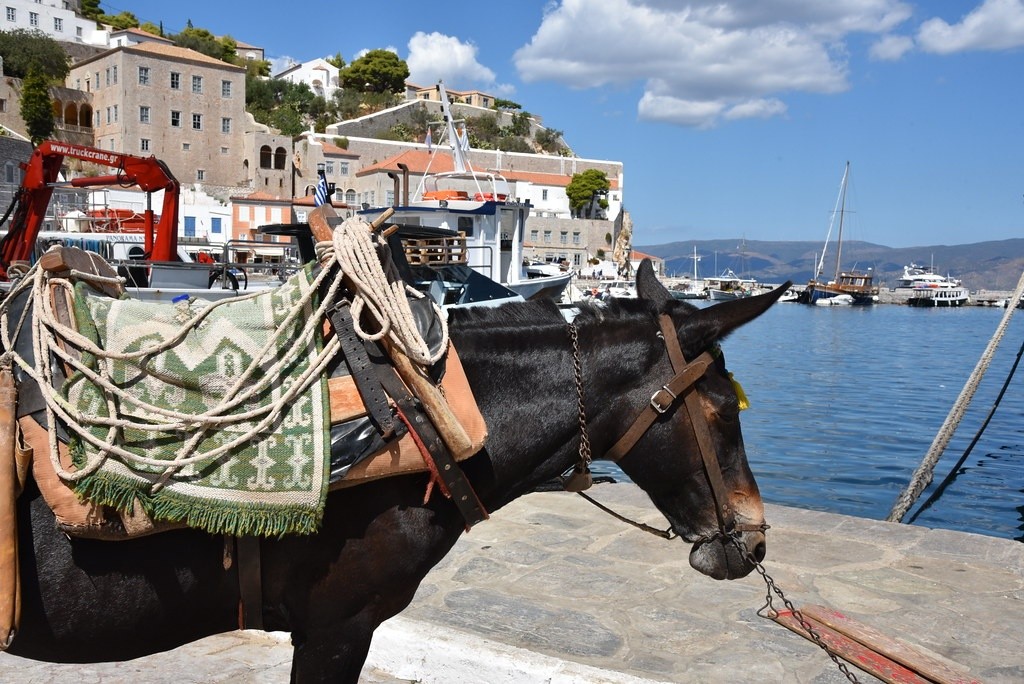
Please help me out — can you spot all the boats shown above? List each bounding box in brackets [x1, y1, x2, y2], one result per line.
[815, 294, 854, 307]
[906, 287, 969, 308]
[778, 291, 798, 301]
[584, 279, 638, 301]
[896, 253, 961, 287]
[124, 81, 575, 304]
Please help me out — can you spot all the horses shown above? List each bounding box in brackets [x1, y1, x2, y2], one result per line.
[1, 256, 793, 684]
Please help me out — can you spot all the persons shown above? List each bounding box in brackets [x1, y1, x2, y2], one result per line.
[599, 269, 602, 279]
[592, 269, 596, 280]
[578, 269, 581, 278]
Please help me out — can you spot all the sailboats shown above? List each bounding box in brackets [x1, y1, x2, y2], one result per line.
[673, 245, 708, 298]
[800, 160, 885, 305]
[711, 234, 753, 300]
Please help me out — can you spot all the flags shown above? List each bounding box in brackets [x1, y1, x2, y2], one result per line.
[425, 127, 432, 149]
[460, 128, 470, 151]
[314, 175, 327, 207]
